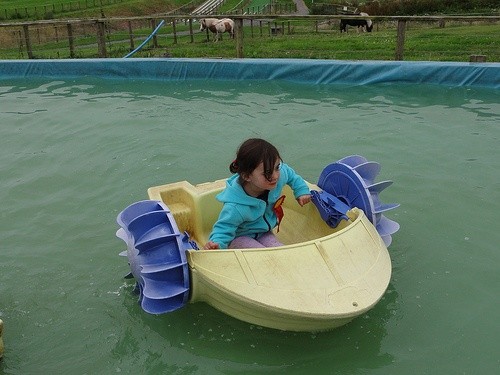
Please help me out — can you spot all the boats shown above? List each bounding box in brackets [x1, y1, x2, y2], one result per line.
[119, 151, 403, 336]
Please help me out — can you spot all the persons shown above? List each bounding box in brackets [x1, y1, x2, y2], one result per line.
[205, 138, 311, 249]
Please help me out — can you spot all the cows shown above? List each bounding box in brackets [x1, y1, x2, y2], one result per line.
[199, 18, 234, 42]
[340, 12, 373, 33]
